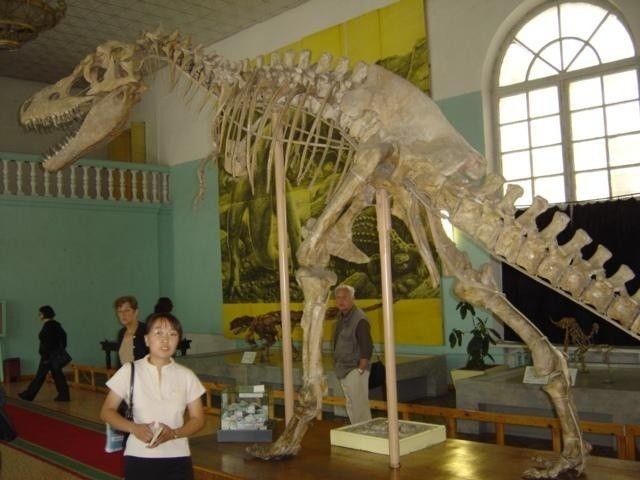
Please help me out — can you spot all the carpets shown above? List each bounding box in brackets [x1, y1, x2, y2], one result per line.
[5, 396, 123, 479]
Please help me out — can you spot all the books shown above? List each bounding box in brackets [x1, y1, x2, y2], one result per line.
[147, 423, 167, 447]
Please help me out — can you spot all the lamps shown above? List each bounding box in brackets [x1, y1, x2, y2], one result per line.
[0, 1, 67, 53]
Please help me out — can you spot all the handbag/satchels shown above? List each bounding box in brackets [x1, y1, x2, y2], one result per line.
[369, 361, 385, 390]
[122, 361, 135, 447]
[39, 344, 72, 372]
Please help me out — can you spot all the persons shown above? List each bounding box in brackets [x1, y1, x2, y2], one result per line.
[155, 296, 173, 313]
[98, 312, 207, 480]
[326, 284, 378, 423]
[113, 294, 152, 371]
[18, 304, 73, 402]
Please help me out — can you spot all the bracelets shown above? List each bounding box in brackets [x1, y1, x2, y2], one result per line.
[170, 429, 179, 440]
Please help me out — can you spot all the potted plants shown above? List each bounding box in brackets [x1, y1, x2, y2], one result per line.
[447, 301, 511, 384]
